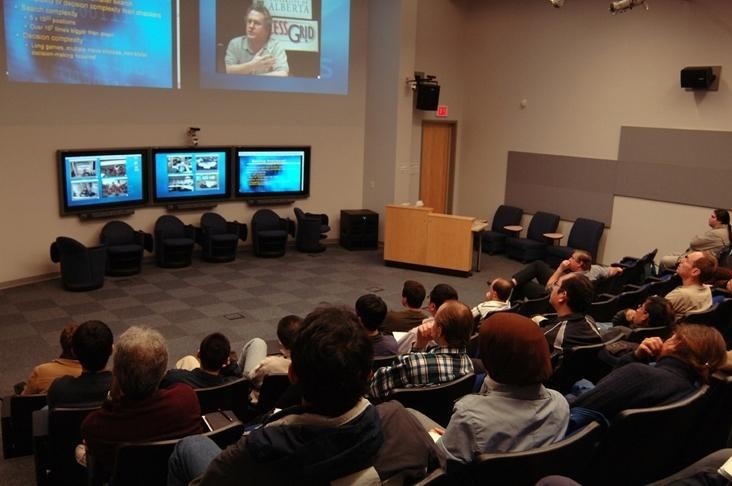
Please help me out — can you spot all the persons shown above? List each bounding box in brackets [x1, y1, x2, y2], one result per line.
[225, 3, 289, 77]
[0, 207, 732, 486]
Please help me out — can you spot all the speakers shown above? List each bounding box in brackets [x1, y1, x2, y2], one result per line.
[341, 209, 378, 251]
[680, 66, 716, 88]
[416, 84, 440, 110]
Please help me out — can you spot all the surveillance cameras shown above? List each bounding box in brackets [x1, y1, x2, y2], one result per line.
[608, 0, 648, 15]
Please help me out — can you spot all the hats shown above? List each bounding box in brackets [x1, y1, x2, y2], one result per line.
[480, 312, 553, 383]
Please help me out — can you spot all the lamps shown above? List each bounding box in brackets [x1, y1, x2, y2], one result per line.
[610, 0, 650, 14]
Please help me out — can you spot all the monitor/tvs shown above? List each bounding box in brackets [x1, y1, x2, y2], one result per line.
[233, 144, 311, 201]
[57, 147, 150, 217]
[149, 145, 233, 206]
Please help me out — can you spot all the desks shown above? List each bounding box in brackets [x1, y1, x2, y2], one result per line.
[384, 203, 490, 274]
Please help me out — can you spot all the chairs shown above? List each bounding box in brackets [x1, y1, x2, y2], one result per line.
[293, 207, 330, 254]
[152, 213, 198, 269]
[98, 220, 154, 278]
[48, 235, 106, 292]
[535, 216, 604, 264]
[474, 205, 523, 254]
[505, 211, 560, 264]
[251, 209, 296, 257]
[200, 213, 248, 264]
